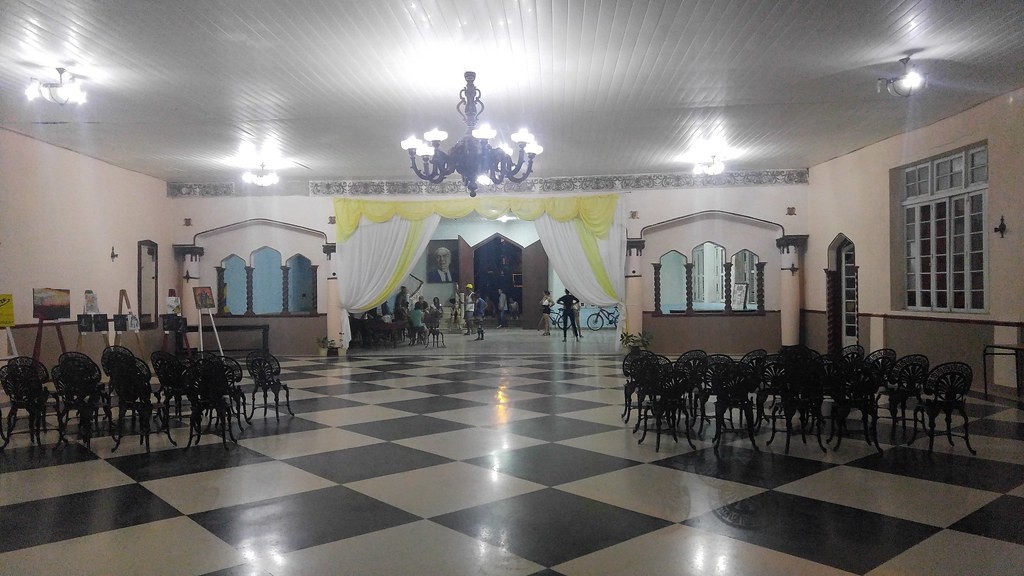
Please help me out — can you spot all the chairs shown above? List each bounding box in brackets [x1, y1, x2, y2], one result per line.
[622, 349, 975, 454]
[0, 345, 296, 455]
[350, 315, 447, 349]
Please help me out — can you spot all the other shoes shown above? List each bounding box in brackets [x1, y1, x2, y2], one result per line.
[543, 332, 550, 335]
[497, 324, 508, 328]
[576, 337, 578, 341]
[562, 339, 566, 342]
[475, 338, 484, 341]
[464, 333, 470, 335]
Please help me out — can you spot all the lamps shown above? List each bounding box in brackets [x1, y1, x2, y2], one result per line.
[886, 58, 930, 98]
[402, 72, 544, 199]
[27, 67, 89, 105]
[242, 164, 278, 188]
[693, 155, 725, 176]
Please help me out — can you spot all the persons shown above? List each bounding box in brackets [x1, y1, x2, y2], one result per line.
[539, 291, 555, 336]
[557, 289, 583, 343]
[484, 296, 520, 321]
[464, 284, 476, 335]
[428, 247, 457, 282]
[496, 288, 508, 328]
[473, 294, 488, 341]
[360, 286, 443, 345]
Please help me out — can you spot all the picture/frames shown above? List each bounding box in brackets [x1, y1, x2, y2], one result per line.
[426, 239, 461, 284]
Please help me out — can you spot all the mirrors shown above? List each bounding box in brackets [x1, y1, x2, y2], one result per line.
[138, 240, 159, 330]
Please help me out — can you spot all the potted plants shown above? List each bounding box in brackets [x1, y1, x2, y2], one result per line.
[618, 331, 653, 354]
[317, 336, 338, 356]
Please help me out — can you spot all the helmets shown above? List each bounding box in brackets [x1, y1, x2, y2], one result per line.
[466, 284, 473, 288]
